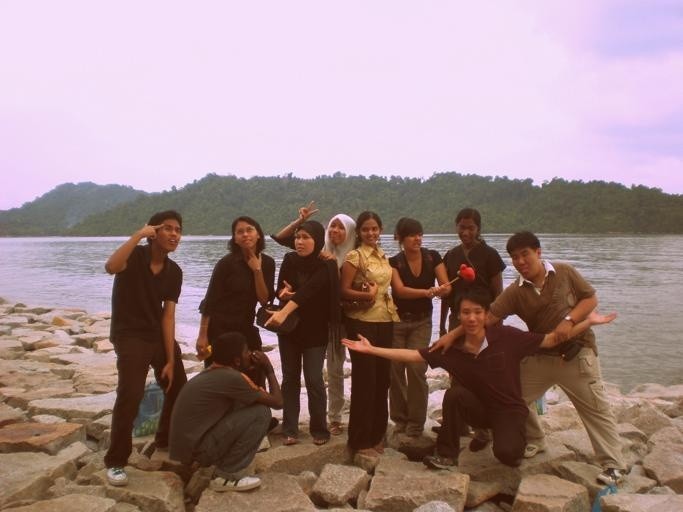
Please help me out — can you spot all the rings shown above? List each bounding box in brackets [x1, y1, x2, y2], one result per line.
[252, 352, 256, 357]
[431, 292, 433, 296]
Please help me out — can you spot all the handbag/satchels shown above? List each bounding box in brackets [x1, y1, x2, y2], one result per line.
[332, 283, 375, 312]
[256, 304, 300, 335]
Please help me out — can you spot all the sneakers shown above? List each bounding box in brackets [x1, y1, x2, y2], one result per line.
[106, 466, 128, 485]
[422, 454, 460, 473]
[470, 437, 488, 452]
[597, 466, 623, 486]
[209, 472, 261, 492]
[405, 421, 425, 437]
[524, 442, 547, 459]
[392, 423, 407, 433]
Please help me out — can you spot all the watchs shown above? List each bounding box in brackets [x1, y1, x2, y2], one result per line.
[564, 314, 577, 326]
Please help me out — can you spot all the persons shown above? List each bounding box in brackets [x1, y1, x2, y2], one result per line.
[338, 286, 616, 465]
[387, 217, 453, 437]
[265, 220, 331, 445]
[167, 330, 282, 494]
[432, 208, 507, 453]
[270, 201, 359, 435]
[341, 210, 401, 457]
[428, 230, 631, 484]
[104, 210, 189, 485]
[194, 217, 276, 379]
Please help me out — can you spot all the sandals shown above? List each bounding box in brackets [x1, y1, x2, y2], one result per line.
[283, 435, 299, 446]
[330, 421, 345, 437]
[313, 433, 332, 446]
